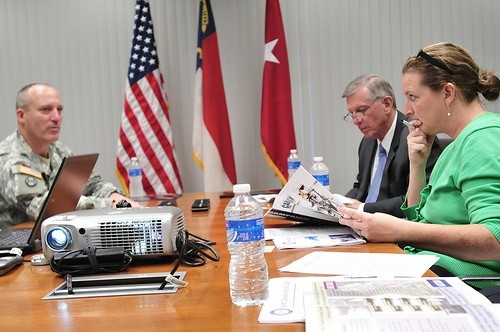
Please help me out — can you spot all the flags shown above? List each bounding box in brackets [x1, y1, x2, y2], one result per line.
[260, 0, 297, 185]
[191, 0, 237, 192]
[116, 0, 184, 197]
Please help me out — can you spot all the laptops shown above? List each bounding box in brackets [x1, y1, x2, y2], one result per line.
[0, 153, 100, 254]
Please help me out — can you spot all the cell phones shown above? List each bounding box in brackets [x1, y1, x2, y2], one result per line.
[159, 200, 176, 207]
[191, 199, 210, 210]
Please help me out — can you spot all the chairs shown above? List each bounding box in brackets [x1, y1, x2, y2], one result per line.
[437, 131, 453, 150]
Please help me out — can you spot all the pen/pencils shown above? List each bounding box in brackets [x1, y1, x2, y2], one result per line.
[400, 119, 416, 130]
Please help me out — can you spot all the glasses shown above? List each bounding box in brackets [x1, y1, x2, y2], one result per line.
[416, 49, 456, 76]
[343, 97, 379, 122]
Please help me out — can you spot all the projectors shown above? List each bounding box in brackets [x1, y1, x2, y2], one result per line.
[40, 206, 186, 265]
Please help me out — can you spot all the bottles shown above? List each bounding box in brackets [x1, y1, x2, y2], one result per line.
[224, 184, 270, 308]
[287, 149, 301, 182]
[129, 157, 143, 198]
[310, 157, 330, 193]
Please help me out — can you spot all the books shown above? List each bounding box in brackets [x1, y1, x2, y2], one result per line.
[263, 165, 352, 226]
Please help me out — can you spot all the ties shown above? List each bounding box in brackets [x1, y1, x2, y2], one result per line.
[365, 143, 387, 203]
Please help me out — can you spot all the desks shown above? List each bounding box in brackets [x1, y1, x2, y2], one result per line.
[0, 192, 441, 332]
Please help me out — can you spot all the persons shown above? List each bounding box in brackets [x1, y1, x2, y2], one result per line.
[337, 42, 500, 292]
[0, 84, 141, 228]
[342, 75, 444, 249]
